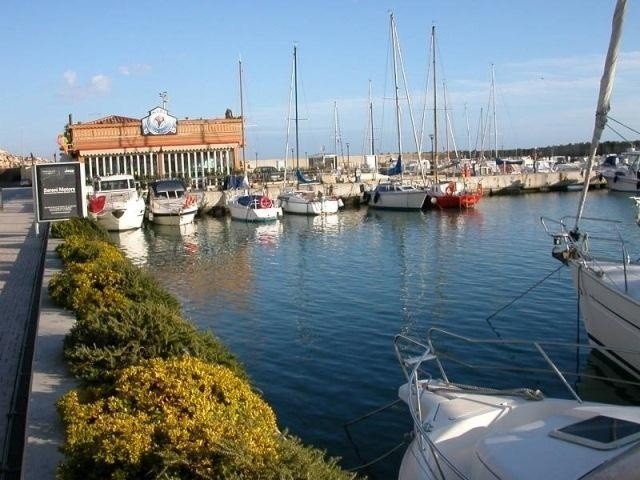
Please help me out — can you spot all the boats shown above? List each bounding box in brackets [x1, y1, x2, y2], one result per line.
[86, 174, 146, 232]
[146, 181, 198, 226]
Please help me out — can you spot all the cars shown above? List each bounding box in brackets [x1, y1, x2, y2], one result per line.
[20, 179, 31, 186]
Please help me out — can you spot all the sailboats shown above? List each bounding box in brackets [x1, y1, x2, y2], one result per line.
[363, 11, 640, 211]
[541, 0, 639, 378]
[274, 44, 346, 215]
[393, 321, 640, 479]
[225, 61, 283, 220]
